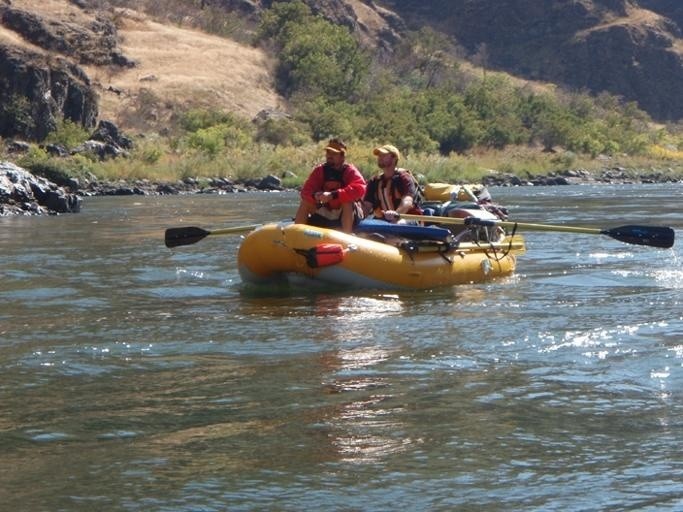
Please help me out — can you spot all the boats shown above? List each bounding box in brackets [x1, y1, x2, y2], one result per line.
[236, 179, 517, 292]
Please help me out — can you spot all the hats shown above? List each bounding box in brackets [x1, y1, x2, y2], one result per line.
[374, 144, 399, 157]
[322, 138, 347, 156]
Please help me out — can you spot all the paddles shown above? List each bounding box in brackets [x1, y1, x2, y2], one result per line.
[165, 224, 256, 248]
[395, 213, 674, 248]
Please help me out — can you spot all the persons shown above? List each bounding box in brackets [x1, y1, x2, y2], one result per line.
[295, 138, 367, 235]
[363, 145, 425, 246]
